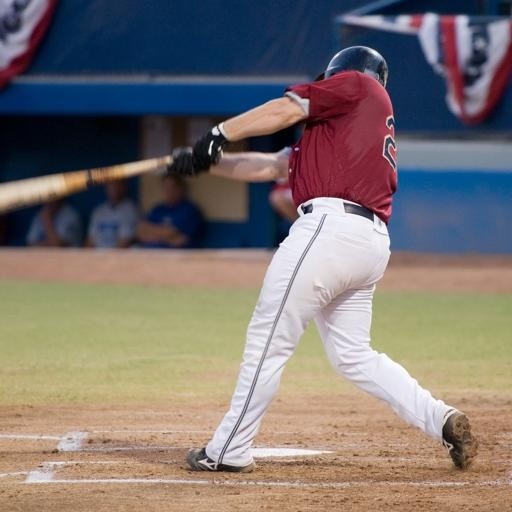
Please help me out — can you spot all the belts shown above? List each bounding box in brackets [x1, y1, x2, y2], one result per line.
[303, 200, 375, 221]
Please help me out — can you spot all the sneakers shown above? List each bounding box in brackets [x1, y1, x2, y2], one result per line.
[442, 410, 478, 471]
[187, 447, 256, 472]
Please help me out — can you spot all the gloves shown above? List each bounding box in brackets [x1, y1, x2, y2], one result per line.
[168, 124, 228, 176]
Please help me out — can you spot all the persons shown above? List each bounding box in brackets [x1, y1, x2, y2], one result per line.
[167, 44, 480, 475]
[85, 180, 141, 250]
[129, 174, 205, 249]
[25, 199, 86, 249]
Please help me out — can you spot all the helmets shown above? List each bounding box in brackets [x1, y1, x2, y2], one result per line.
[314, 45, 388, 89]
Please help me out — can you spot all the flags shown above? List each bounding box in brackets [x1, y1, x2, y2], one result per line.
[0, 0, 55, 93]
[343, 12, 511, 125]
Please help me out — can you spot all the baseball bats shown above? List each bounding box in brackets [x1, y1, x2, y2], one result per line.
[0, 156, 177, 215]
